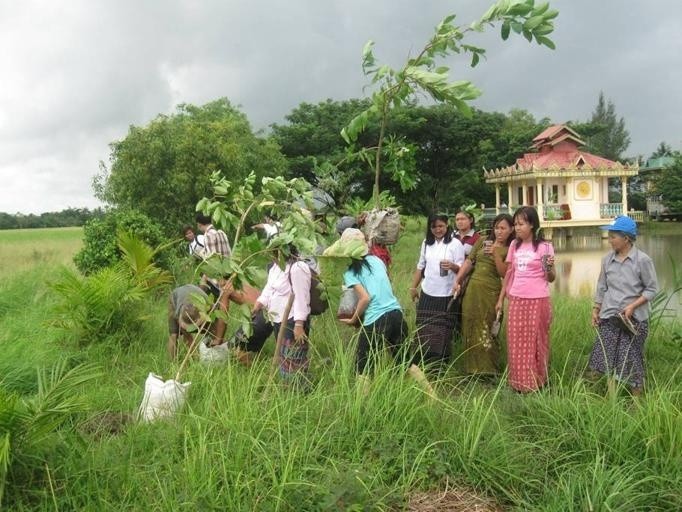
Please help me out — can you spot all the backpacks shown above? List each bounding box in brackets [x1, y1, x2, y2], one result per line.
[289, 260, 330, 315]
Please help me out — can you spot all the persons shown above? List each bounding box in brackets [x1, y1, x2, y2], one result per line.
[168, 212, 329, 383]
[592, 216, 659, 397]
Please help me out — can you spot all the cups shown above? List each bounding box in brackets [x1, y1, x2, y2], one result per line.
[540, 254, 551, 272]
[482, 240, 493, 257]
[439, 260, 448, 277]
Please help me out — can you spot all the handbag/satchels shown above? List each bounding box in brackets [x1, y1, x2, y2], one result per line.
[420, 267, 426, 278]
[364, 207, 401, 245]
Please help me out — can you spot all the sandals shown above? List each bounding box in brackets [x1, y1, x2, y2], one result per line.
[610, 311, 638, 337]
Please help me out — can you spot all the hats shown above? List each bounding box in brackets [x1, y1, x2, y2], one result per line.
[178, 304, 205, 332]
[318, 216, 368, 257]
[598, 215, 637, 236]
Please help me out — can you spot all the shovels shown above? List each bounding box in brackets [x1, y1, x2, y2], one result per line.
[446, 285, 460, 312]
[490, 309, 502, 338]
